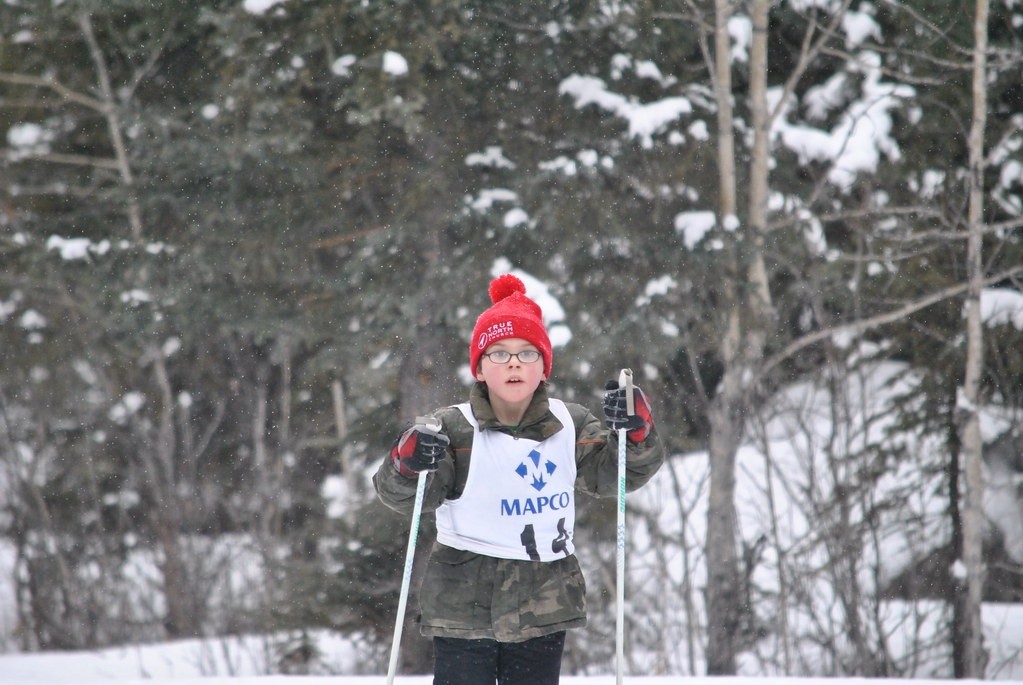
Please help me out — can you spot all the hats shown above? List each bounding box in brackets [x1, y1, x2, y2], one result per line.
[470, 274, 552, 381]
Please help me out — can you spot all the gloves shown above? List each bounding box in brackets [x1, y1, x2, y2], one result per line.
[390, 424, 451, 479]
[603, 379, 653, 444]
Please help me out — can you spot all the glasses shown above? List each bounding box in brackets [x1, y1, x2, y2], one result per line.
[482, 350, 542, 364]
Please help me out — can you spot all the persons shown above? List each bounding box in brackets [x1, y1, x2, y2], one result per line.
[372, 274, 665, 685]
